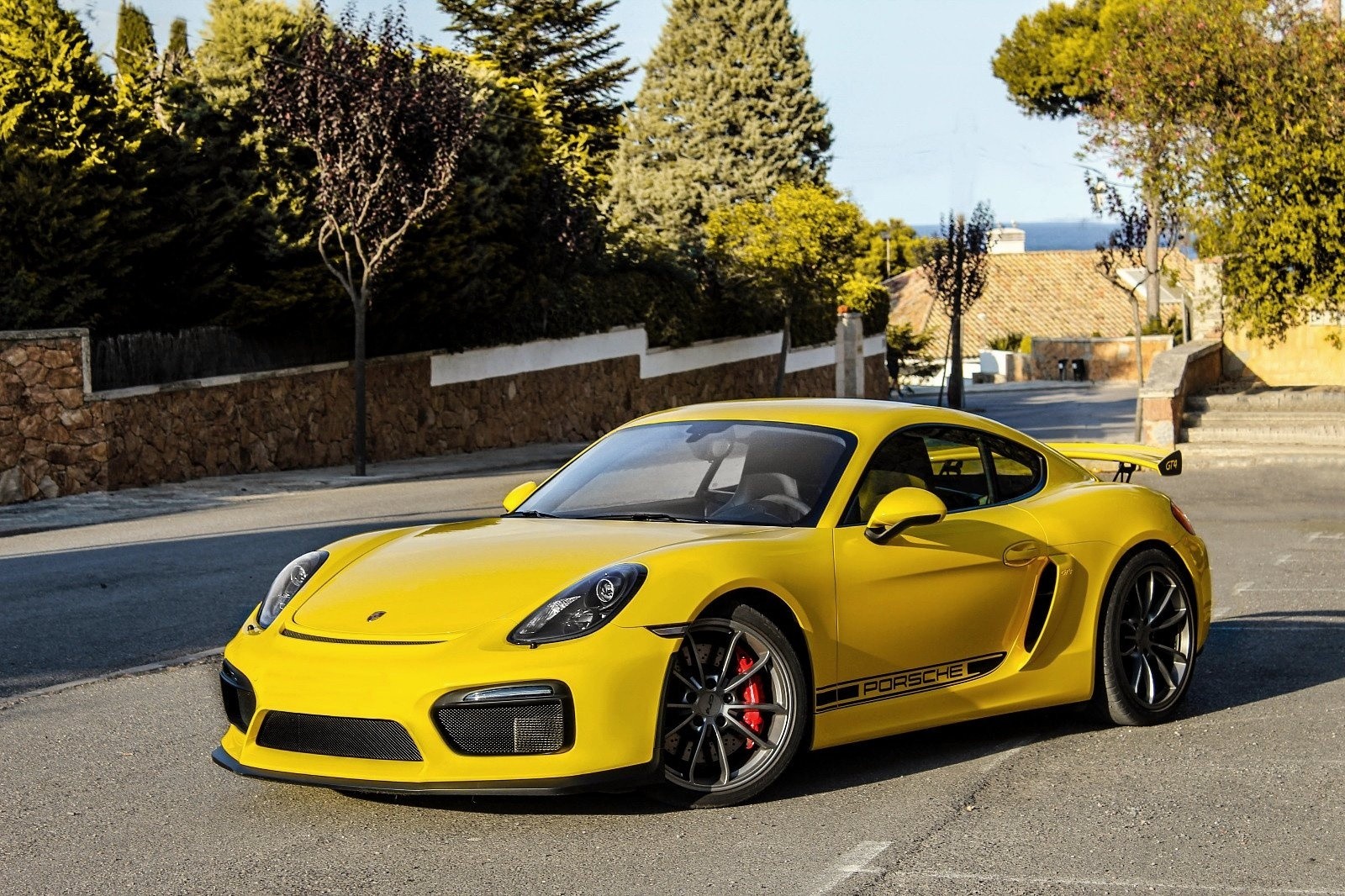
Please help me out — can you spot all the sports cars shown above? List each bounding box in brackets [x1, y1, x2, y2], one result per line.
[212, 395, 1212, 808]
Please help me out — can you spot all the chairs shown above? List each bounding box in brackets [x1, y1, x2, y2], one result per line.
[703, 427, 933, 528]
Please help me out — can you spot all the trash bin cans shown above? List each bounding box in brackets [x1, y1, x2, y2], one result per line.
[1072, 359, 1085, 382]
